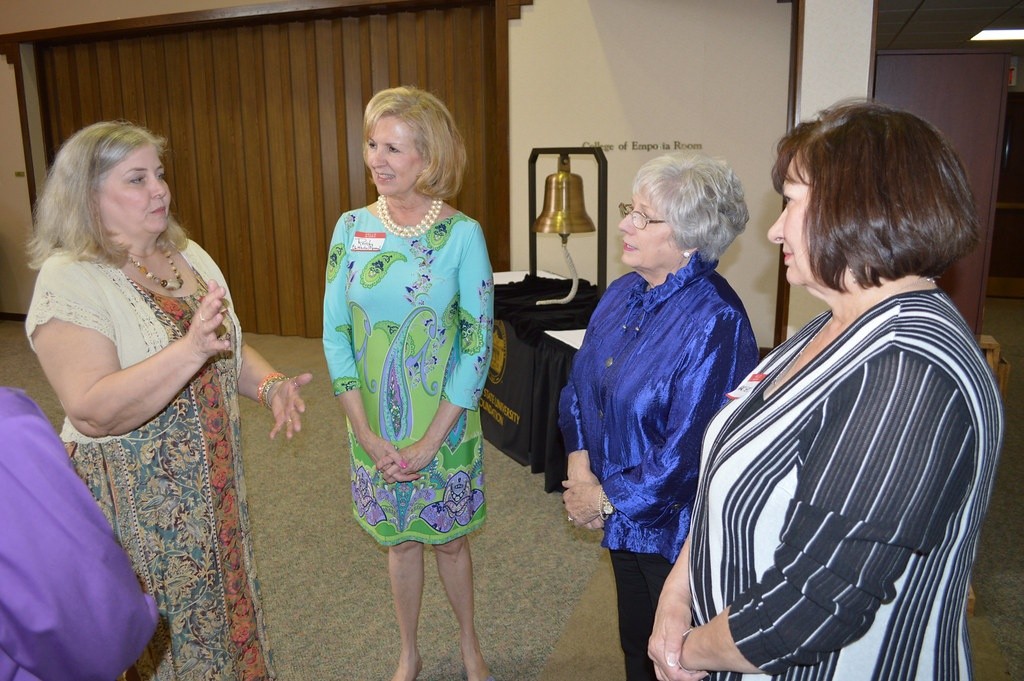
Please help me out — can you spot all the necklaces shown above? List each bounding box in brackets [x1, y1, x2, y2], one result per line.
[126, 238, 183, 290]
[377, 195, 443, 237]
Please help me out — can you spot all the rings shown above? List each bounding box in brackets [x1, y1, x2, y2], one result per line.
[287, 419, 292, 424]
[200, 314, 206, 321]
[292, 376, 300, 389]
[568, 516, 573, 522]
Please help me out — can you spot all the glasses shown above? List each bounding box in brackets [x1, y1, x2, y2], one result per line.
[619, 202, 666, 229]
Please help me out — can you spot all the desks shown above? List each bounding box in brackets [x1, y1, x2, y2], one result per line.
[479, 269, 599, 493]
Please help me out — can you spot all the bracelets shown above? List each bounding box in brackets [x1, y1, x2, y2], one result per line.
[257, 373, 286, 410]
[598, 487, 609, 521]
[676, 628, 697, 674]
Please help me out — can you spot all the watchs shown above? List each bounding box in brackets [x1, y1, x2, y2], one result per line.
[603, 493, 614, 515]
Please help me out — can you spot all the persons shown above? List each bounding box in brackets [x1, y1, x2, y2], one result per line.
[24, 120, 314, 681]
[557, 153, 757, 681]
[646, 98, 1003, 681]
[0, 386, 159, 681]
[321, 87, 493, 681]
